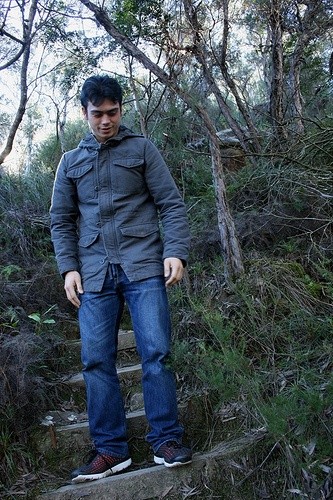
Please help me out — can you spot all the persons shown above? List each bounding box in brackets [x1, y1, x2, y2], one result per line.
[50, 74, 192, 482]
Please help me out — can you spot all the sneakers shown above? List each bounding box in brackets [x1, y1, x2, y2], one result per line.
[70, 452, 132, 482]
[154, 441, 192, 468]
